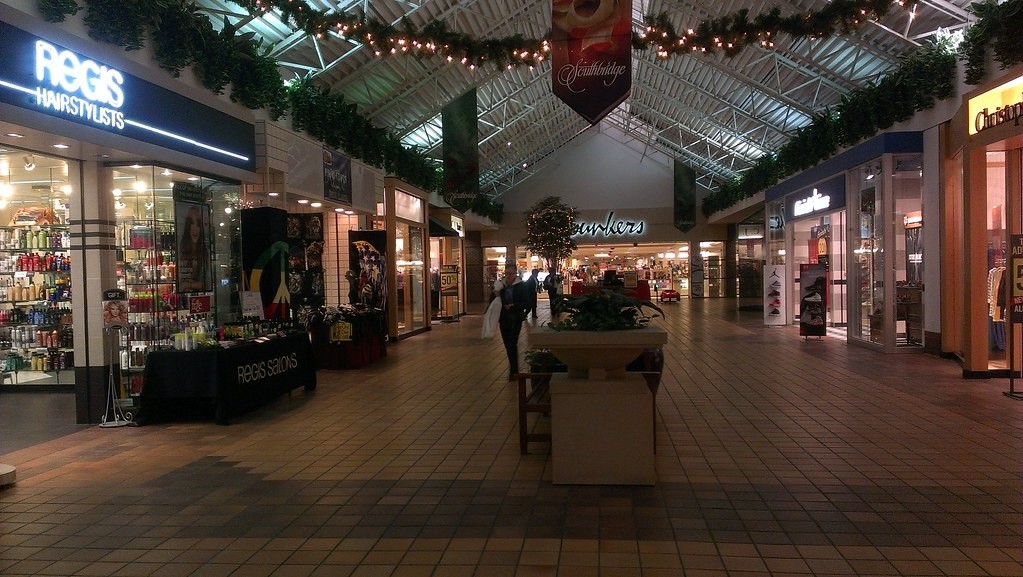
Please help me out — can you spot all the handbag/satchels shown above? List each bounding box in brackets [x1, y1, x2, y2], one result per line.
[543, 285, 550, 290]
[555, 282, 563, 295]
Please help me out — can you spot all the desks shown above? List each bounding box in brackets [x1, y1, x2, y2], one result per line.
[310, 311, 387, 370]
[134, 332, 314, 426]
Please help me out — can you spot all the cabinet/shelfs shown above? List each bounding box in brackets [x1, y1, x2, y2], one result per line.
[0, 223, 218, 386]
[870, 283, 921, 349]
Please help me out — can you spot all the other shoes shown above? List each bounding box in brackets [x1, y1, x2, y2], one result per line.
[532, 314, 538, 318]
[509, 373, 518, 379]
[860, 255, 872, 306]
[896, 279, 921, 319]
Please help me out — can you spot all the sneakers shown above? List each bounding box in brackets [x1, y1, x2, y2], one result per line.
[804, 293, 822, 302]
[771, 281, 781, 287]
[805, 307, 822, 314]
[806, 316, 823, 325]
[770, 299, 781, 306]
[805, 282, 821, 291]
[769, 308, 781, 316]
[768, 290, 781, 298]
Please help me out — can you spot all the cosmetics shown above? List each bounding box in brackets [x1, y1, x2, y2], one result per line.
[0, 205, 295, 373]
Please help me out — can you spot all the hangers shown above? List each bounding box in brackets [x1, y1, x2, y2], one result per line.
[992, 258, 1007, 272]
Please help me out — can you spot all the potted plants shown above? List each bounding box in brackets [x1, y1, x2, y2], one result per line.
[524, 286, 669, 373]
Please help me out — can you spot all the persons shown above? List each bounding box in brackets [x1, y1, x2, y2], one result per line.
[180, 205, 209, 289]
[490, 263, 528, 379]
[526, 269, 542, 318]
[578, 268, 587, 282]
[543, 267, 564, 315]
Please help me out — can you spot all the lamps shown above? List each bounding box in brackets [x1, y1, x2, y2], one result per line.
[22, 153, 36, 171]
[865, 166, 882, 179]
